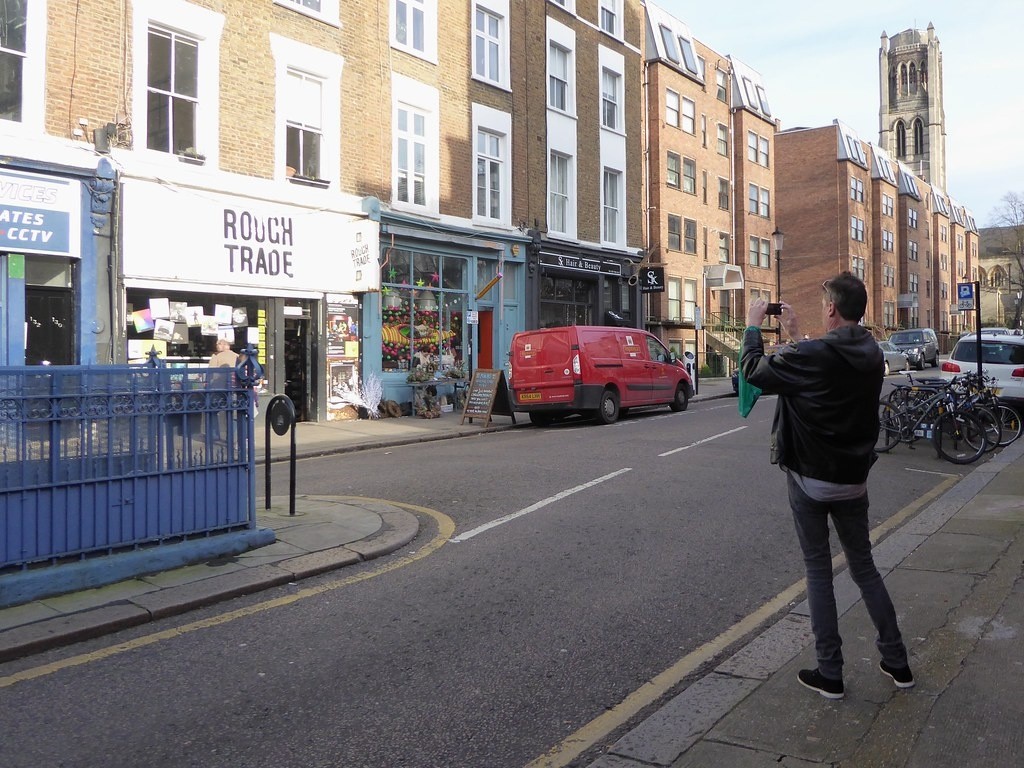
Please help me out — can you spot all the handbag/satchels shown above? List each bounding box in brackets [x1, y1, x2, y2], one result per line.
[737, 325, 764, 420]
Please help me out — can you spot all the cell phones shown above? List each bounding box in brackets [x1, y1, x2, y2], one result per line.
[765, 303, 782, 315]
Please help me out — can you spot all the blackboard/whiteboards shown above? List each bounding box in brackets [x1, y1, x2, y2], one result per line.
[463, 369, 502, 419]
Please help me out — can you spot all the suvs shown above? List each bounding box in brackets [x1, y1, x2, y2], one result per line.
[974, 327, 1012, 356]
[942, 332, 1023, 423]
[888, 328, 940, 371]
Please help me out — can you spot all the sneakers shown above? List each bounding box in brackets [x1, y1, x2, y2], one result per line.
[879, 661, 915, 688]
[798, 668, 844, 698]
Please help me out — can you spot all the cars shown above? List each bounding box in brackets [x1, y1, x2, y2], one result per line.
[876, 340, 910, 377]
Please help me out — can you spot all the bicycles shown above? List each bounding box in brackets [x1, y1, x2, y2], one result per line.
[873, 370, 1023, 465]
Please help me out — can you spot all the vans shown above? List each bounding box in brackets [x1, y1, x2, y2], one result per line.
[508, 324, 694, 426]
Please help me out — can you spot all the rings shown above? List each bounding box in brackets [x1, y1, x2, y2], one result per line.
[748, 304, 753, 306]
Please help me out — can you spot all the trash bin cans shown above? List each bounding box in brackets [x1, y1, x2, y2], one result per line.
[683, 351, 696, 396]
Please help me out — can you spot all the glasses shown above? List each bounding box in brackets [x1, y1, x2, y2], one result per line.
[821, 280, 835, 302]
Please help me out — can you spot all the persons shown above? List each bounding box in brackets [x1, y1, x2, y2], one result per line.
[203, 340, 258, 444]
[1014, 326, 1023, 336]
[803, 334, 809, 340]
[332, 375, 354, 392]
[738, 272, 916, 699]
[332, 320, 356, 342]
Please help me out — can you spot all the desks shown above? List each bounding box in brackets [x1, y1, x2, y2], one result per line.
[408, 379, 465, 417]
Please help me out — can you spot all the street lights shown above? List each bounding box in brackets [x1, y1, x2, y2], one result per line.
[961, 274, 968, 331]
[1014, 298, 1019, 327]
[1007, 263, 1012, 294]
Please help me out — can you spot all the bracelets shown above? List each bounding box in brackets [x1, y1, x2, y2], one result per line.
[745, 322, 761, 327]
